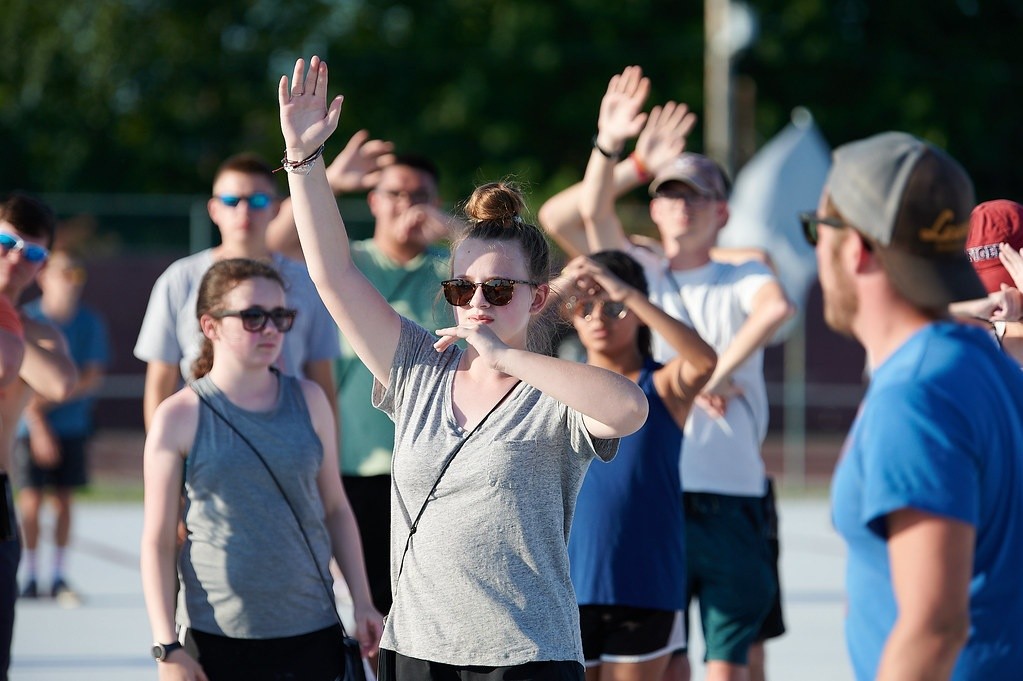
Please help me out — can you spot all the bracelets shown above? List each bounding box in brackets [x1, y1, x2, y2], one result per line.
[591, 135, 620, 162]
[989, 321, 1007, 351]
[628, 149, 656, 184]
[271, 142, 325, 176]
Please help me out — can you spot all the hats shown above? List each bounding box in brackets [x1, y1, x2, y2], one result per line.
[968, 199, 1023, 296]
[830, 132, 990, 307]
[649, 150, 727, 204]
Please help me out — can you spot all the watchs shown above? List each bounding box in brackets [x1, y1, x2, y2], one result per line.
[152, 641, 182, 662]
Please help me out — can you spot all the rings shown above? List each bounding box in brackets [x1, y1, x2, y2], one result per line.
[290, 92, 302, 97]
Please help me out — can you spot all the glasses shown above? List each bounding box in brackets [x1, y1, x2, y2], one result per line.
[799, 210, 877, 255]
[209, 193, 283, 210]
[1, 232, 56, 261]
[441, 278, 540, 308]
[217, 307, 297, 333]
[572, 298, 635, 316]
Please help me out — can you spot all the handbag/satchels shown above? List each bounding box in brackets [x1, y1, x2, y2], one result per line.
[343, 636, 369, 681]
[375, 649, 396, 681]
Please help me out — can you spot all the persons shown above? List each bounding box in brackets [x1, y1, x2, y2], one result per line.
[539, 63, 795, 681]
[0, 193, 106, 681]
[133, 149, 470, 681]
[278, 54, 650, 681]
[142, 257, 384, 681]
[799, 131, 1023, 681]
[541, 248, 718, 681]
